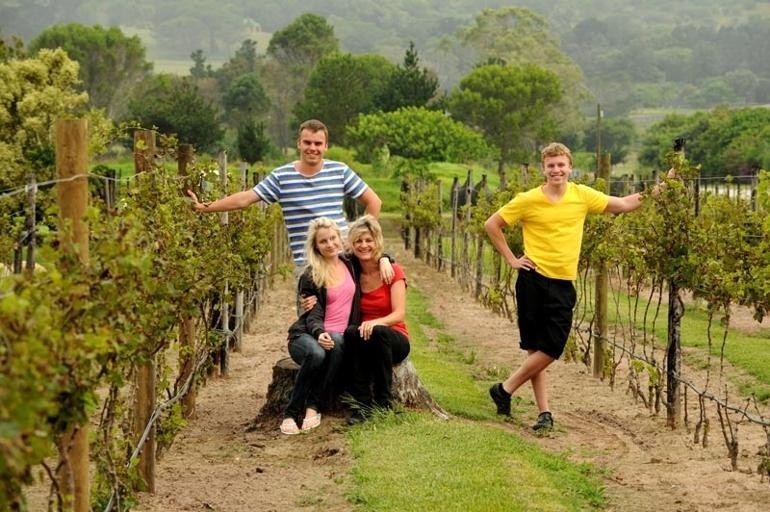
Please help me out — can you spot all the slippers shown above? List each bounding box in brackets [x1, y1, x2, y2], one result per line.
[279, 421, 297, 435]
[301, 414, 321, 430]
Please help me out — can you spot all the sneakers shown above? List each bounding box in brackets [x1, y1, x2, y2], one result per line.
[489, 383, 511, 415]
[532, 411, 552, 429]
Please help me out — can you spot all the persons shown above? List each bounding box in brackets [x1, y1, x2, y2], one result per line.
[296, 210, 412, 427]
[185, 118, 384, 316]
[481, 140, 681, 435]
[278, 215, 396, 438]
